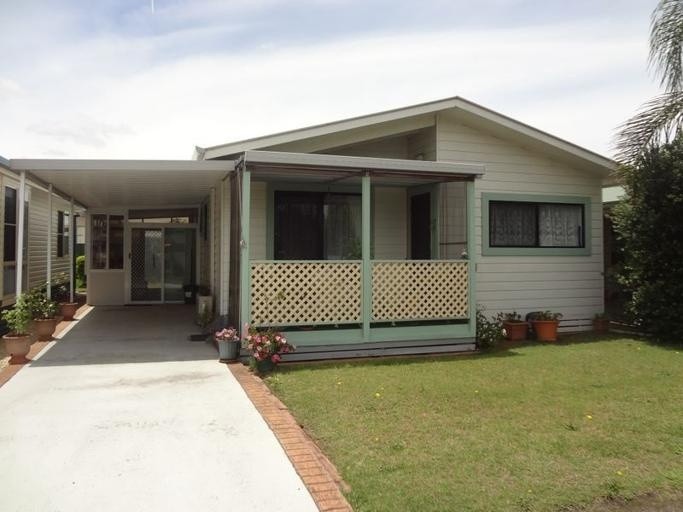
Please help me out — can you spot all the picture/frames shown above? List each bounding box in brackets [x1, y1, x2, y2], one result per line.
[216, 337, 239, 362]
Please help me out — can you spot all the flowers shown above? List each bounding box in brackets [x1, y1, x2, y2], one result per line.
[243, 320, 295, 373]
[215, 326, 240, 343]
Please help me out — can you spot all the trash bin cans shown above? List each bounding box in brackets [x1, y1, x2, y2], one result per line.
[182, 284, 200, 304]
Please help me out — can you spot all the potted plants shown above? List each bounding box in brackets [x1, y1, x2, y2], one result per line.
[24, 284, 58, 341]
[1, 297, 32, 364]
[504, 311, 529, 341]
[527, 309, 563, 342]
[53, 272, 78, 321]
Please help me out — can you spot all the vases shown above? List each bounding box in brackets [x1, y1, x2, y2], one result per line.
[257, 360, 272, 372]
